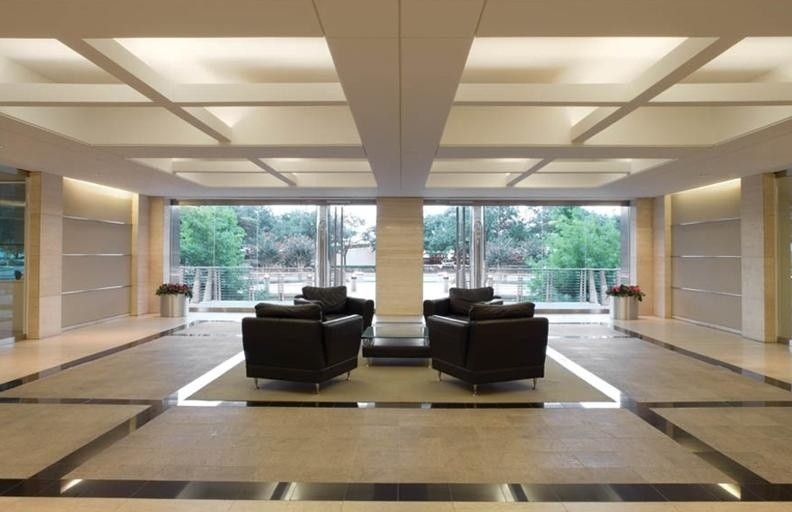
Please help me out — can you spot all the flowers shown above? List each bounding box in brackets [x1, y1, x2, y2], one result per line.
[605, 285, 645, 301]
[156, 284, 192, 298]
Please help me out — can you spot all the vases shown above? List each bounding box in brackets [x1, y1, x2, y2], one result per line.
[160, 295, 189, 317]
[610, 296, 638, 320]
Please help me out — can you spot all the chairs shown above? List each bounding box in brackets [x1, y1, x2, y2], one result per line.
[427, 315, 548, 396]
[243, 314, 363, 394]
[295, 295, 374, 330]
[424, 296, 500, 318]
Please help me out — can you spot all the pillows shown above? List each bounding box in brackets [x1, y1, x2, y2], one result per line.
[256, 303, 322, 320]
[469, 303, 535, 320]
[449, 287, 493, 315]
[303, 286, 346, 315]
